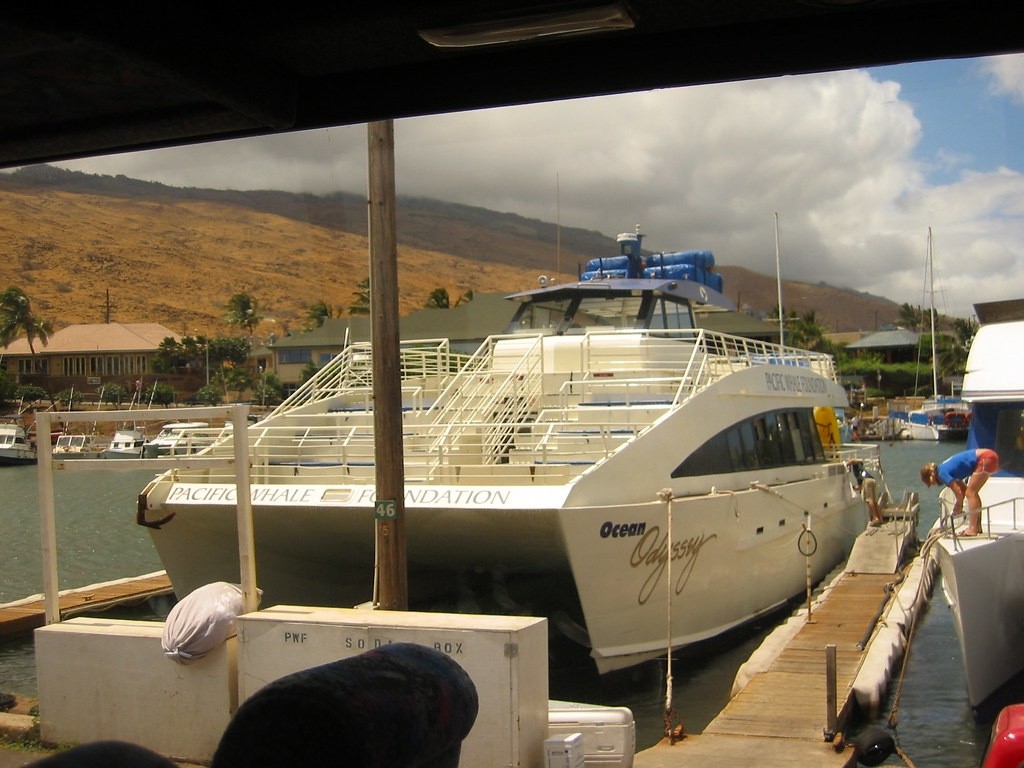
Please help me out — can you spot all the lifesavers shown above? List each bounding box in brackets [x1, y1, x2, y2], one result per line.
[983, 704, 1024, 768]
[945, 412, 971, 427]
[813, 406, 840, 450]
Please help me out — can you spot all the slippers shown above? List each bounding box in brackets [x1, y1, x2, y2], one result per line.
[959, 530, 977, 537]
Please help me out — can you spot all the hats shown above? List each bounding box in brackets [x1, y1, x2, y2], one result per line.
[921, 464, 933, 488]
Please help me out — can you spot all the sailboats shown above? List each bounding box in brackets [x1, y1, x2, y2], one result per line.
[889, 227, 972, 440]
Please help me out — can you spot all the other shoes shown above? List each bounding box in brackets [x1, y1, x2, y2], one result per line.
[869, 521, 881, 525]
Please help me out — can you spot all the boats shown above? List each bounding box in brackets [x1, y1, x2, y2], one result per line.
[0, 416, 210, 465]
[934, 299, 1024, 705]
[132, 225, 877, 675]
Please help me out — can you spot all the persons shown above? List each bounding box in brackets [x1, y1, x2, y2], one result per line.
[920, 448, 999, 535]
[851, 414, 859, 430]
[847, 460, 883, 526]
